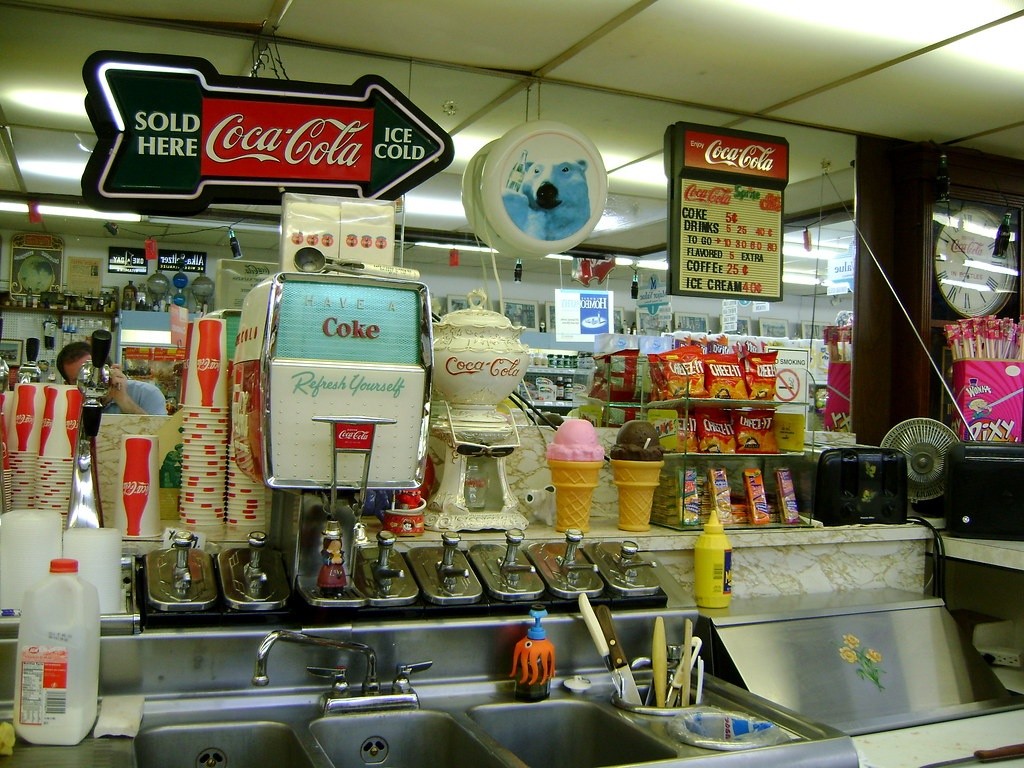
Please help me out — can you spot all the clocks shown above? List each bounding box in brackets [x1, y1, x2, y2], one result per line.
[934, 206, 1018, 322]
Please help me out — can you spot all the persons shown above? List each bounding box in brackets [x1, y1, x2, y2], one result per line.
[56, 342, 168, 415]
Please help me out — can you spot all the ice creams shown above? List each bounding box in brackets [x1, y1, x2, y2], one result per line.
[546, 417, 607, 532]
[610, 419, 665, 532]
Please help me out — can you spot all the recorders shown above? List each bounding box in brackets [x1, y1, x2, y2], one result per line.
[823, 171, 1024, 543]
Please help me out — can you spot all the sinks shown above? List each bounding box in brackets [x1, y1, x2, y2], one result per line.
[463, 696, 679, 768]
[132, 720, 317, 768]
[308, 710, 512, 768]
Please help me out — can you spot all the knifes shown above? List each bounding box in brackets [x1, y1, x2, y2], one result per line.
[579, 592, 643, 706]
[666, 637, 702, 709]
[920, 743, 1024, 768]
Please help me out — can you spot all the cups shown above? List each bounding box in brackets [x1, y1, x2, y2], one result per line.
[0, 383, 85, 541]
[114, 435, 163, 537]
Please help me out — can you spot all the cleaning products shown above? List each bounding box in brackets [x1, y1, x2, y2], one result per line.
[509, 604, 557, 704]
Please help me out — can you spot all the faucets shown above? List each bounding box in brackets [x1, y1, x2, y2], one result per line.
[250, 629, 382, 694]
[170, 529, 199, 591]
[496, 527, 536, 580]
[435, 530, 471, 580]
[555, 527, 600, 579]
[372, 528, 405, 586]
[611, 540, 658, 578]
[243, 531, 271, 591]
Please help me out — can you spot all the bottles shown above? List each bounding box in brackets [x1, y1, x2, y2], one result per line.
[527, 352, 578, 369]
[14, 558, 101, 746]
[506, 150, 527, 193]
[742, 329, 747, 335]
[464, 460, 487, 511]
[26, 286, 33, 307]
[122, 268, 214, 314]
[621, 320, 646, 335]
[656, 322, 669, 336]
[62, 318, 110, 348]
[694, 509, 733, 608]
[37, 290, 117, 312]
[556, 376, 573, 402]
[539, 318, 546, 331]
[179, 318, 265, 526]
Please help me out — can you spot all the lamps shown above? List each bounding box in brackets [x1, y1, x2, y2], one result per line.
[514, 258, 522, 285]
[879, 418, 960, 504]
[630, 271, 639, 299]
[103, 223, 119, 236]
[229, 227, 242, 260]
